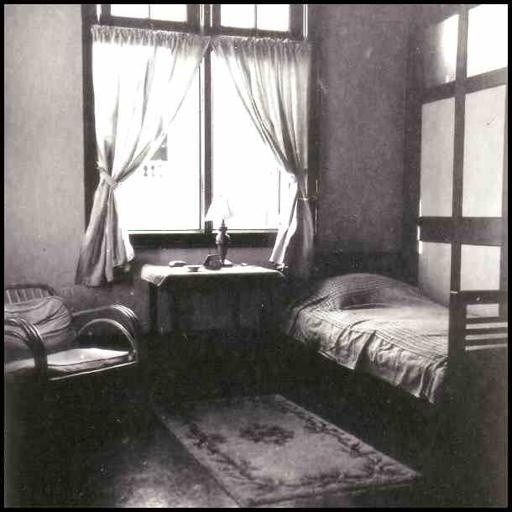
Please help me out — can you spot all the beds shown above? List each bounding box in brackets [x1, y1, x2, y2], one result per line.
[290, 247, 510, 431]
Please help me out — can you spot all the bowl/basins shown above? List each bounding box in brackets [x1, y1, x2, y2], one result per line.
[184, 266, 200, 271]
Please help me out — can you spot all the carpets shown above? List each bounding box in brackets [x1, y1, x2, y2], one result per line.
[153, 389, 427, 509]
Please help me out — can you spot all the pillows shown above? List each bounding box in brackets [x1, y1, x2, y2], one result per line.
[316, 267, 430, 313]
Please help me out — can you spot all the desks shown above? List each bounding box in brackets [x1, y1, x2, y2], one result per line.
[144, 259, 286, 368]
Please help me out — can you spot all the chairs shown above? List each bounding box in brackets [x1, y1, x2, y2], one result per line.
[2, 278, 147, 429]
